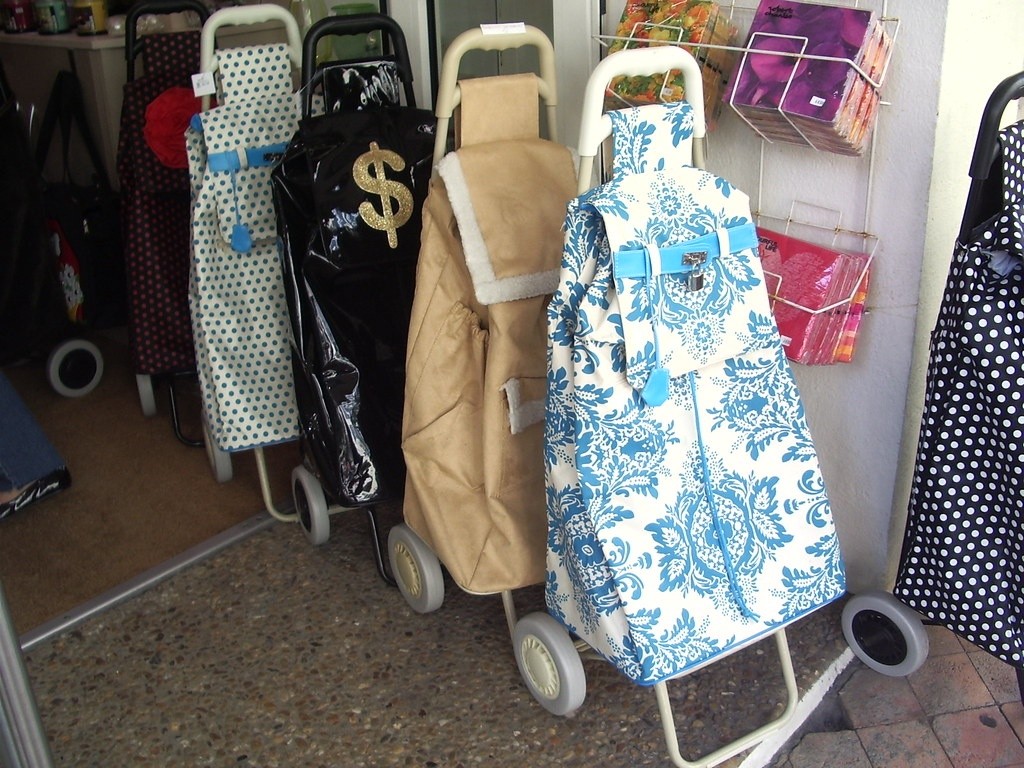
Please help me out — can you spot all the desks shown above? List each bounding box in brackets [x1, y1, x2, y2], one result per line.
[1, 20, 287, 191]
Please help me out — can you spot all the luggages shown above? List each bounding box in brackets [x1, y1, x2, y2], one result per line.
[0, 65, 105, 399]
[893, 77, 1024, 706]
[100, 2, 930, 768]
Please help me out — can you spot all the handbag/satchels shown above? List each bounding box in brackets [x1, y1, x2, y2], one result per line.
[15, 72, 128, 332]
